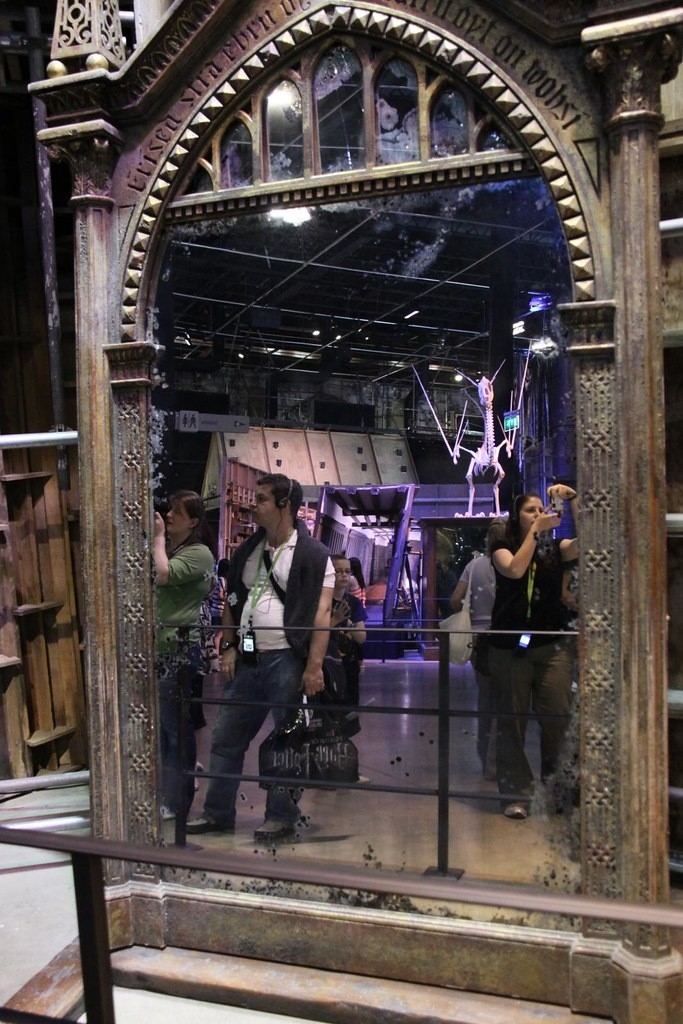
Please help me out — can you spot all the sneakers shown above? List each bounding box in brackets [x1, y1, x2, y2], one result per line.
[254, 815, 308, 841]
[186, 814, 234, 834]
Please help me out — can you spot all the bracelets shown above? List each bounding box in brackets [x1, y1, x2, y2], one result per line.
[350, 622, 356, 627]
[568, 493, 578, 501]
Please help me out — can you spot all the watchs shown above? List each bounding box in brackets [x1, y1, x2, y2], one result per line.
[222, 641, 236, 650]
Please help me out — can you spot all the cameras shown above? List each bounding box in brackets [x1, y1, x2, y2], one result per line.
[153, 495, 171, 521]
[551, 490, 564, 510]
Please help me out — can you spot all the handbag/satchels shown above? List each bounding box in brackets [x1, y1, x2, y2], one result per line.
[313, 657, 350, 704]
[438, 557, 473, 666]
[259, 685, 359, 790]
[468, 635, 498, 677]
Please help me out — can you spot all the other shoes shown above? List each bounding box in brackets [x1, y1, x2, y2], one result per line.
[193, 761, 205, 772]
[503, 801, 527, 818]
[160, 805, 175, 820]
[196, 781, 202, 791]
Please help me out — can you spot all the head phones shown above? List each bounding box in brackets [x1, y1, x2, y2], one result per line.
[509, 494, 522, 528]
[275, 478, 294, 509]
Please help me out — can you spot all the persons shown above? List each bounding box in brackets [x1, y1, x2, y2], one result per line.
[452, 484, 580, 818]
[153, 473, 368, 842]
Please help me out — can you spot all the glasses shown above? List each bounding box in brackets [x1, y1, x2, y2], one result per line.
[336, 569, 353, 576]
